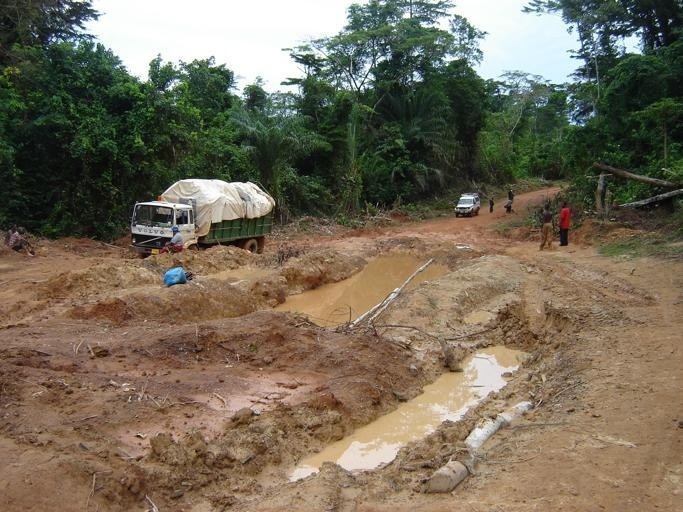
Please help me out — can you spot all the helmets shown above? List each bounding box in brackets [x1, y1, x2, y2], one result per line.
[171, 227, 180, 232]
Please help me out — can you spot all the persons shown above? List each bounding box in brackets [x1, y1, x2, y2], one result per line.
[5, 223, 36, 257]
[489, 197, 494, 213]
[160, 225, 184, 254]
[504, 189, 515, 213]
[539, 202, 555, 251]
[557, 201, 571, 246]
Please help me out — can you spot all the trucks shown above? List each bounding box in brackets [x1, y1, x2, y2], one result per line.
[126, 199, 275, 259]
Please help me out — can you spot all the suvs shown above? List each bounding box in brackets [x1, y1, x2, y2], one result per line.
[452, 191, 481, 218]
